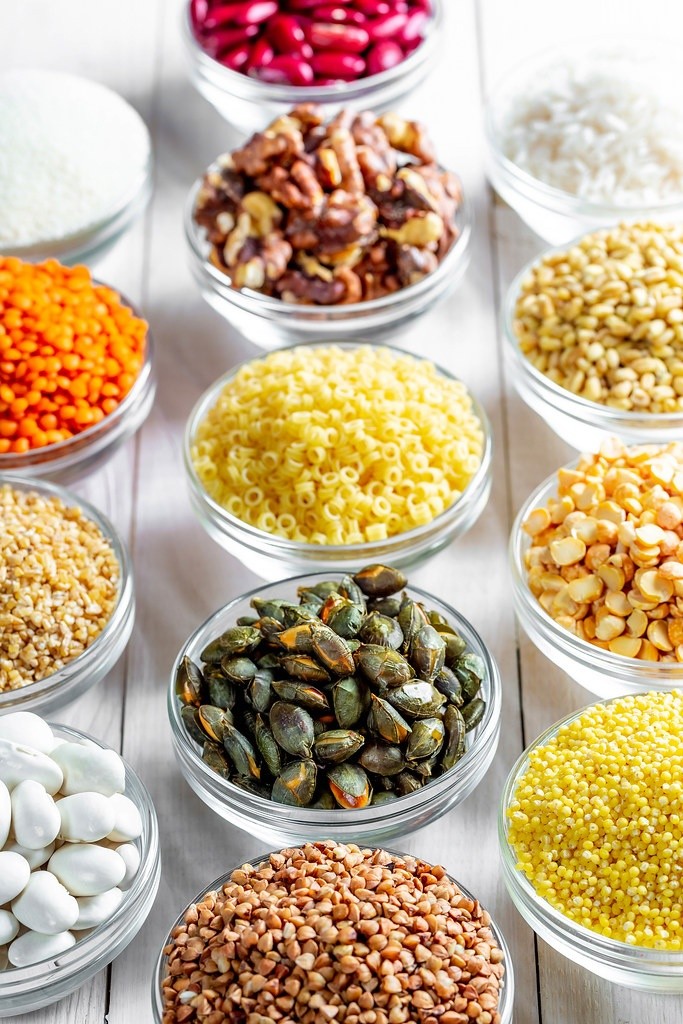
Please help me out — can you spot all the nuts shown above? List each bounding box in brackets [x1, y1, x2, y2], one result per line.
[161, 839, 503, 1024]
[180, 565, 483, 808]
[189, 0, 430, 87]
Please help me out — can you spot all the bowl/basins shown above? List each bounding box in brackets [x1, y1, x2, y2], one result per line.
[499, 220, 683, 452]
[0, 257, 157, 481]
[182, 138, 484, 354]
[180, 341, 493, 583]
[151, 843, 518, 1024]
[164, 569, 503, 850]
[0, 714, 163, 1018]
[0, 68, 153, 264]
[478, 60, 683, 246]
[0, 475, 139, 715]
[174, 0, 445, 137]
[498, 690, 683, 995]
[507, 435, 683, 699]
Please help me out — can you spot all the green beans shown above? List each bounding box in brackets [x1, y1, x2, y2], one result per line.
[0, 255, 148, 452]
[511, 223, 683, 414]
[0, 711, 142, 964]
